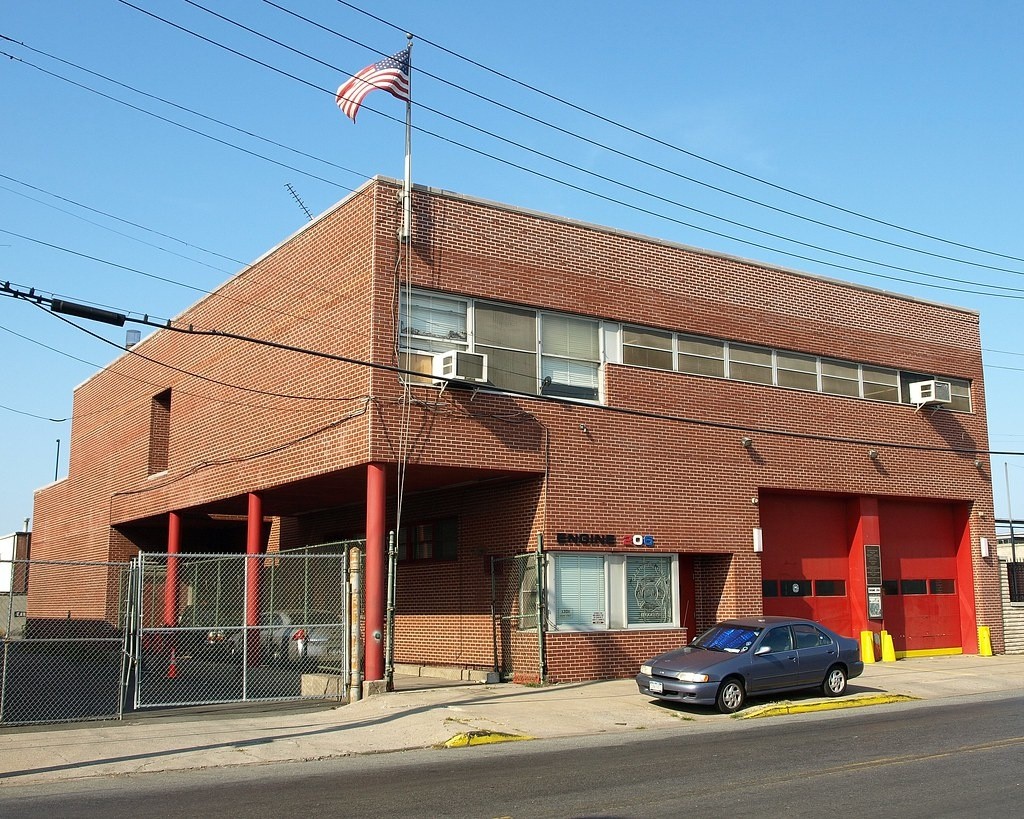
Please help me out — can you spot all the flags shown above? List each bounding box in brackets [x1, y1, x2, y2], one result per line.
[335, 49, 409, 125]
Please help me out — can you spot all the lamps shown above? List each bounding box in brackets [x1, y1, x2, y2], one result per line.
[868, 450, 878, 459]
[740, 437, 752, 447]
[974, 459, 983, 468]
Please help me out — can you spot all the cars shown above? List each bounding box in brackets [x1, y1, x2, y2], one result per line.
[228, 607, 340, 669]
[635, 616, 865, 716]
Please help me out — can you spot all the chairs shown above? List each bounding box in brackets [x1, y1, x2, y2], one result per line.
[768, 630, 786, 652]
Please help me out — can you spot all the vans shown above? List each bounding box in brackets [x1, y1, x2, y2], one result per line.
[23, 617, 132, 667]
[182, 603, 241, 662]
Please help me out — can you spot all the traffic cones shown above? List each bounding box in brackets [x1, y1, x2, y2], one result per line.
[166, 648, 179, 680]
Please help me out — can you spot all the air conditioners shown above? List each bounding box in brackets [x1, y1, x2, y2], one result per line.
[431, 350, 488, 385]
[908, 380, 952, 405]
